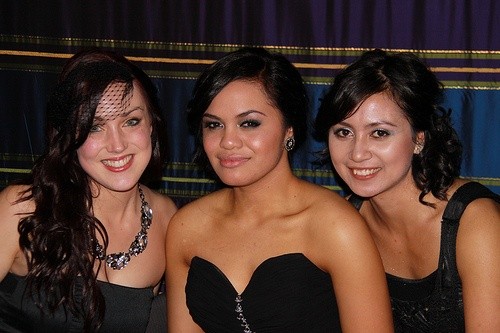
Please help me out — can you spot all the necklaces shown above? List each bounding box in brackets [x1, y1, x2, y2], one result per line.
[87, 180, 154, 271]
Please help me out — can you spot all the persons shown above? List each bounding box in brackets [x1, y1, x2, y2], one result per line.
[313, 48, 500, 332]
[160, 45, 396, 332]
[0, 50, 178, 330]
